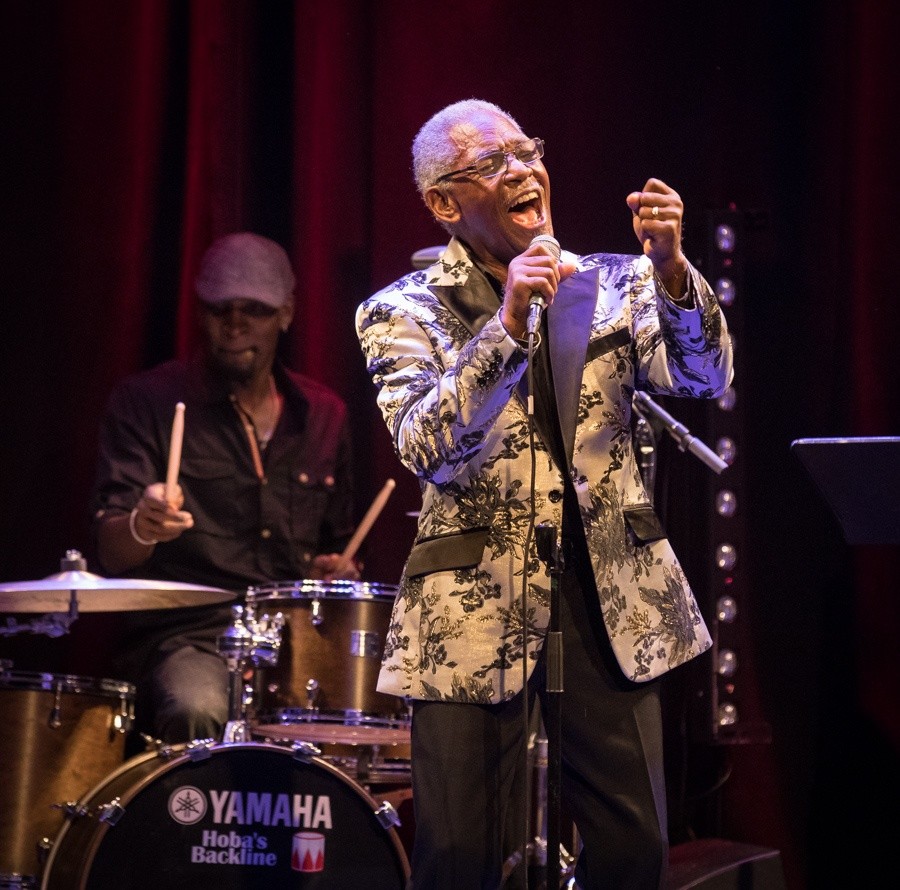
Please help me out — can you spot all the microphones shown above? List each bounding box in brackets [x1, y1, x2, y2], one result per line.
[525, 234, 560, 335]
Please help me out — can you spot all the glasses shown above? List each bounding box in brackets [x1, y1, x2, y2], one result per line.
[434, 137, 544, 183]
[203, 302, 277, 318]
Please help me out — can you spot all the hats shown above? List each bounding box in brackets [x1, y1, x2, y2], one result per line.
[193, 234, 296, 307]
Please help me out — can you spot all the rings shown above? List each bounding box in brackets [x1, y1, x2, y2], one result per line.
[652, 207, 659, 220]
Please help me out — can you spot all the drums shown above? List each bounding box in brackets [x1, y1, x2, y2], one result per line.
[0, 677, 135, 877]
[40, 741, 415, 890]
[243, 580, 410, 745]
[304, 742, 413, 785]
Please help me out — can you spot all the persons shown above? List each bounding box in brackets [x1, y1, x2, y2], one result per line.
[355, 101, 737, 890]
[95, 231, 380, 750]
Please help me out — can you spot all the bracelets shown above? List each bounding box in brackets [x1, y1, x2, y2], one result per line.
[128, 507, 160, 547]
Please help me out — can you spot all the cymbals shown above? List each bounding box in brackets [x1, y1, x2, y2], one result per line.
[1, 566, 239, 614]
